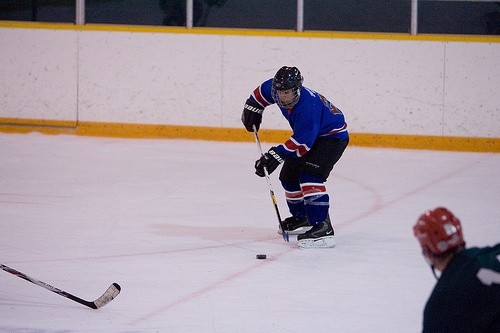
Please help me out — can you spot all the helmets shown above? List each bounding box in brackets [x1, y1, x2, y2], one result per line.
[271, 66, 304, 110]
[413, 208, 464, 266]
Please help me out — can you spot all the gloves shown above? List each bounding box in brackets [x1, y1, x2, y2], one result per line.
[254, 146, 286, 177]
[241, 99, 264, 132]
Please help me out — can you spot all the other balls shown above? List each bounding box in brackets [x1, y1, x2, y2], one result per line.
[256, 254, 266, 259]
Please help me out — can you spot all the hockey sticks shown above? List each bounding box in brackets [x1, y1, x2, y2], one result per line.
[0, 263, 121, 309]
[252, 123, 289, 243]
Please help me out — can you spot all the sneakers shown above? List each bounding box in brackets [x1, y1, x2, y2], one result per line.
[297, 213, 336, 249]
[278, 215, 313, 235]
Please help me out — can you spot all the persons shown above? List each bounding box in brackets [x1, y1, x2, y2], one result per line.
[413, 206, 500, 333]
[241, 66, 348, 249]
